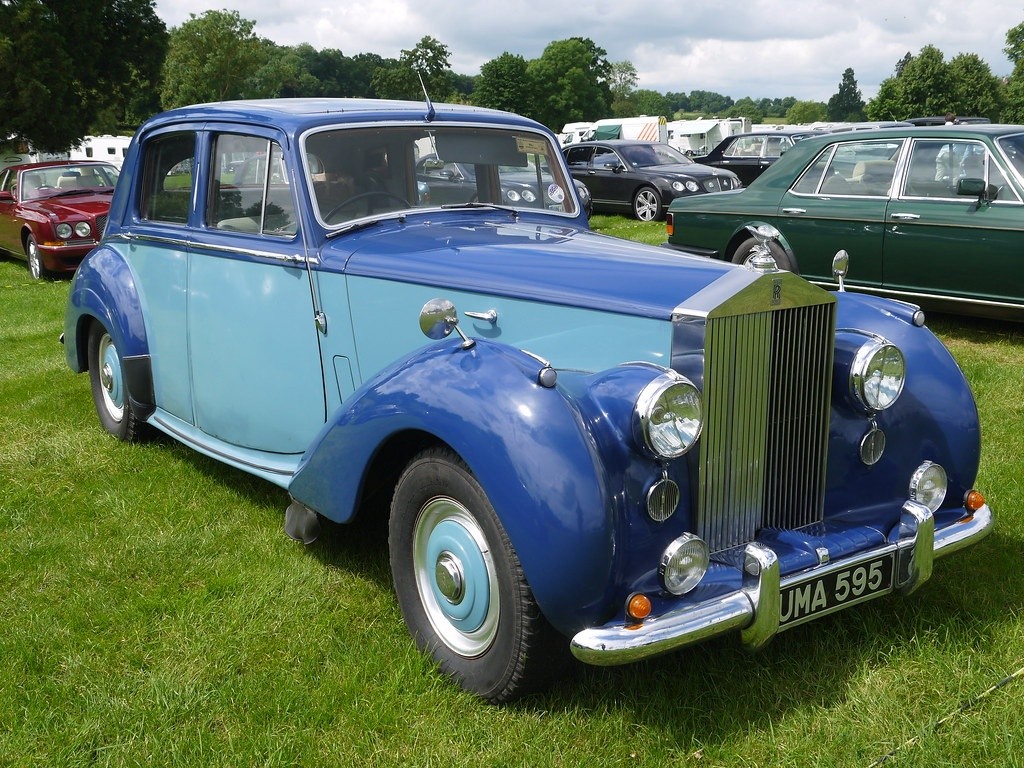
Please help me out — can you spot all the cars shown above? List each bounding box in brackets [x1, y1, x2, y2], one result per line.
[691, 114, 992, 188]
[60, 101, 991, 705]
[234, 136, 592, 229]
[661, 124, 1024, 324]
[0, 161, 121, 279]
[541, 140, 742, 222]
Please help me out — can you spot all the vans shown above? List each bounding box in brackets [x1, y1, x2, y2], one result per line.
[557, 115, 667, 150]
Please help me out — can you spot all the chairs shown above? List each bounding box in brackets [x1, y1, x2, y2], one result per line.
[216, 202, 356, 238]
[767, 141, 782, 158]
[10, 173, 81, 189]
[909, 163, 954, 197]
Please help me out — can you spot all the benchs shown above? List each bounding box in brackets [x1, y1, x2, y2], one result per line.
[804, 160, 896, 197]
[734, 143, 761, 156]
[150, 186, 350, 222]
